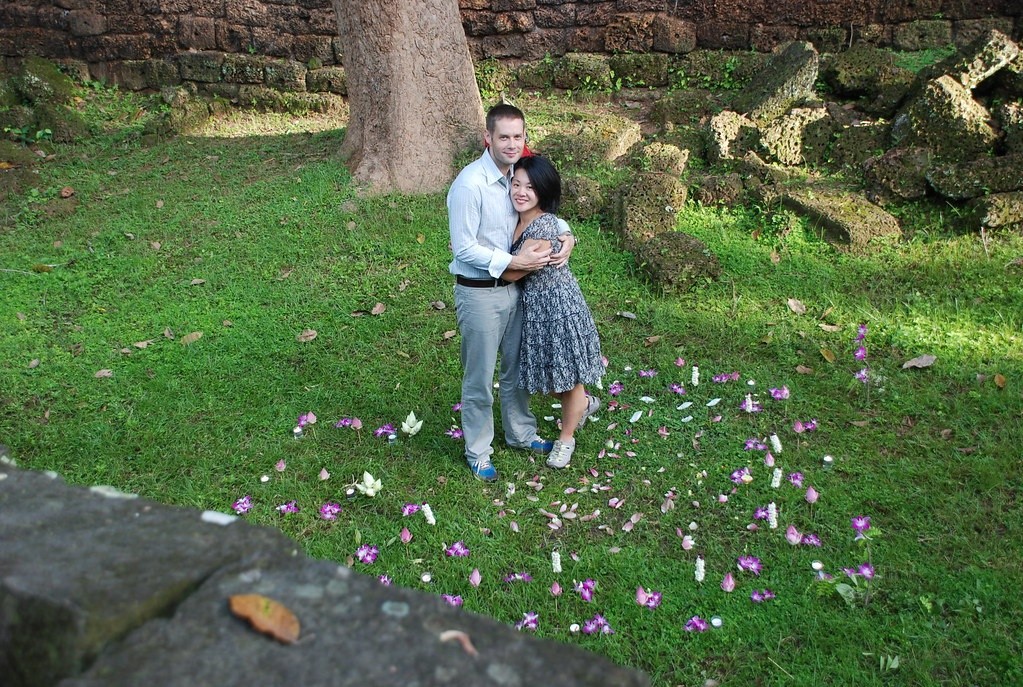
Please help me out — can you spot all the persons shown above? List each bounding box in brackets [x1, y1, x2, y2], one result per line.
[447, 104, 575, 482]
[500, 156, 607, 469]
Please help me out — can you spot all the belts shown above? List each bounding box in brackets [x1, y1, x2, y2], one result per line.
[456, 276, 512, 287]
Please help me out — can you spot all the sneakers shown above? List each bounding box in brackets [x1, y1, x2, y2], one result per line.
[505, 435, 554, 455]
[576, 395, 601, 428]
[468, 459, 499, 483]
[546, 437, 576, 468]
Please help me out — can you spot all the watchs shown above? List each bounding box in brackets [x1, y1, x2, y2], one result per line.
[568, 234, 578, 246]
